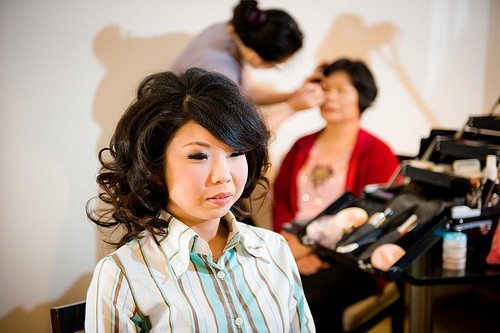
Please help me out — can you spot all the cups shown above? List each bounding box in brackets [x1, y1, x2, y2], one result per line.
[444, 234, 466, 269]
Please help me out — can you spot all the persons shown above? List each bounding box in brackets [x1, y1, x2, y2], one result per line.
[85, 58, 405, 333]
[172, 0, 335, 227]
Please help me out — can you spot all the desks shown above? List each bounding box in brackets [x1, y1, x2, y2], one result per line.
[315, 245, 500, 286]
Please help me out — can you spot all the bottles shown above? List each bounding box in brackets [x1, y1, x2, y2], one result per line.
[482, 155, 498, 183]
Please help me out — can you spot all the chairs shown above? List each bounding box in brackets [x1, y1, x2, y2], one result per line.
[340, 155, 413, 333]
